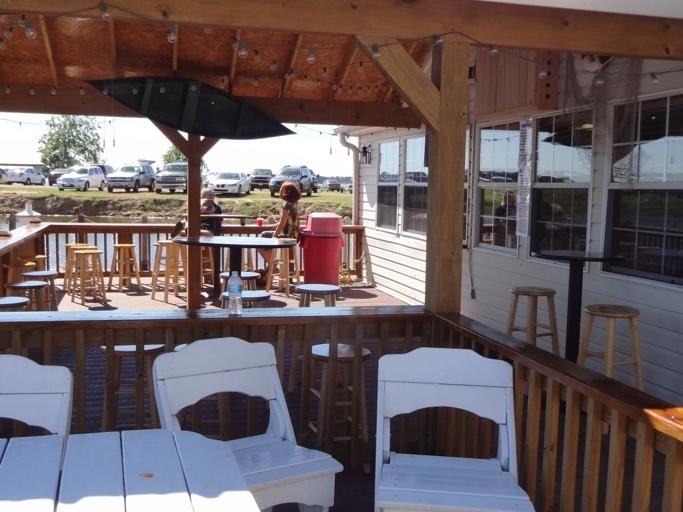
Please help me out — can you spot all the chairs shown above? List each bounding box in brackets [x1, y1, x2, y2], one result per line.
[153, 334, 345, 512]
[373, 348, 533, 512]
[1, 354, 74, 438]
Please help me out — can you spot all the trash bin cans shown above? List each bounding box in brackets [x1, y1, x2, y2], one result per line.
[299, 212, 345, 286]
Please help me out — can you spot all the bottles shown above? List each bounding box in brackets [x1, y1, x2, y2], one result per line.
[228, 272, 245, 315]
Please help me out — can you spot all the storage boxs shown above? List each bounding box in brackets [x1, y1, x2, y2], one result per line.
[304, 210, 343, 237]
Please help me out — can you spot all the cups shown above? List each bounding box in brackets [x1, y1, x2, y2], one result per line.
[256, 218, 264, 227]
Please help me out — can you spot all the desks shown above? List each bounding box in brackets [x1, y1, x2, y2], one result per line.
[176, 213, 254, 298]
[3, 428, 263, 512]
[531, 250, 625, 363]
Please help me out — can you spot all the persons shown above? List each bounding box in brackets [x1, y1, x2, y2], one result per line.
[257, 181, 301, 278]
[201, 188, 223, 285]
[186, 199, 215, 233]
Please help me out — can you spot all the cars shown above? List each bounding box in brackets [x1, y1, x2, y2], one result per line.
[49, 168, 69, 186]
[322, 179, 341, 192]
[91, 164, 115, 177]
[55, 166, 105, 192]
[4, 167, 46, 187]
[207, 172, 250, 196]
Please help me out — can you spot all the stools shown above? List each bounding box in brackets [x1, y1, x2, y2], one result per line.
[579, 304, 641, 385]
[101, 345, 162, 427]
[306, 343, 370, 451]
[0, 235, 188, 311]
[265, 237, 341, 306]
[506, 285, 561, 359]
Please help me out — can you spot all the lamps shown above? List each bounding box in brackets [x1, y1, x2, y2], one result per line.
[359, 144, 370, 165]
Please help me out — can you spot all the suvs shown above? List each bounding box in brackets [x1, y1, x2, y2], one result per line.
[309, 169, 319, 193]
[154, 162, 188, 194]
[247, 168, 276, 191]
[268, 165, 313, 198]
[104, 164, 156, 194]
